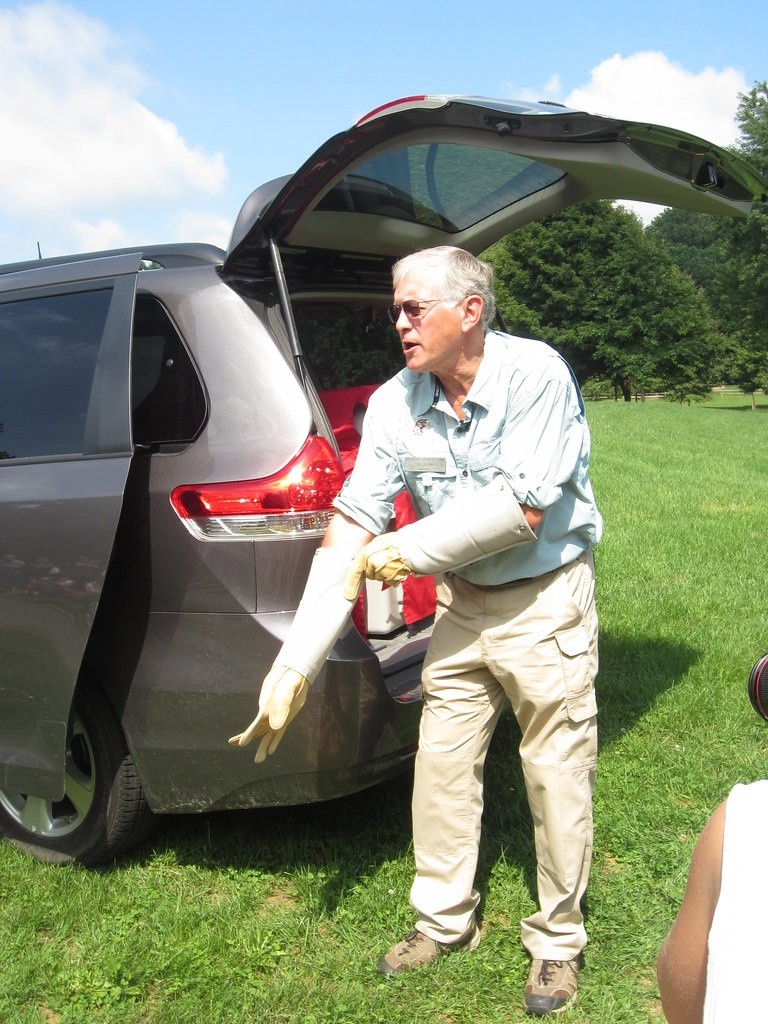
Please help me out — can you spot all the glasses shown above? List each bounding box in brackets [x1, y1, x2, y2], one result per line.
[385, 296, 443, 322]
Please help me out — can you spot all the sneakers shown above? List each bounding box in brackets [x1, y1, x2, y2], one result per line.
[378, 925, 483, 976]
[523, 957, 580, 1014]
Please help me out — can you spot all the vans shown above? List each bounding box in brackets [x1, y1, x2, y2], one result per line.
[0, 89, 768, 869]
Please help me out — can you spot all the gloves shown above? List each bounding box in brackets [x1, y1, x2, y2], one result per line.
[228, 547, 369, 764]
[343, 475, 539, 601]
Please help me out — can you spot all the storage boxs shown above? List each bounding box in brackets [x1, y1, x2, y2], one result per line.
[365, 511, 405, 635]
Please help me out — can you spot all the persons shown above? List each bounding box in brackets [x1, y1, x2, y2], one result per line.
[229, 244, 604, 1014]
[656, 780, 768, 1024]
